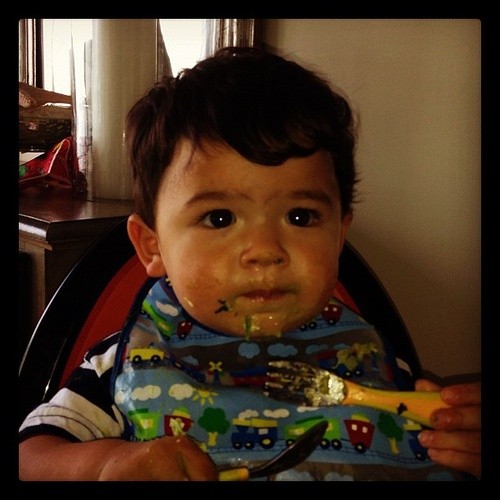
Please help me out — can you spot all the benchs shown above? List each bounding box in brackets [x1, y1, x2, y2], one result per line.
[18, 83, 75, 152]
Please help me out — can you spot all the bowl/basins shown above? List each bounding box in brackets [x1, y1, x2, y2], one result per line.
[19, 152, 45, 180]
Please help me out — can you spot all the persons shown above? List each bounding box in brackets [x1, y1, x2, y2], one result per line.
[19, 42, 482, 484]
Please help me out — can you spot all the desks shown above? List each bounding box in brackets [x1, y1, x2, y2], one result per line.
[18, 196, 135, 327]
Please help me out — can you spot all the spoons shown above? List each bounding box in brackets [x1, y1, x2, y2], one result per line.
[219, 420, 328, 481]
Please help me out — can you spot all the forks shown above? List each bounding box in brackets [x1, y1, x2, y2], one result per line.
[262, 361, 452, 430]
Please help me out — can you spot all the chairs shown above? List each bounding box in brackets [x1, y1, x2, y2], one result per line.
[19, 222, 417, 405]
